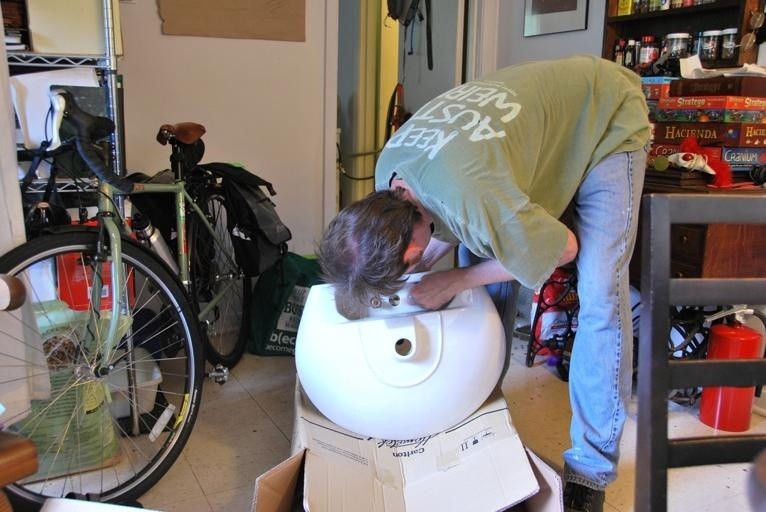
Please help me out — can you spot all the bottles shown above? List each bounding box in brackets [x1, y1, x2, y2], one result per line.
[613, 28, 740, 70]
[131, 213, 180, 278]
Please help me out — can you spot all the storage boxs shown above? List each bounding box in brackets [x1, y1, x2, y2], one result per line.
[249, 373, 566, 512]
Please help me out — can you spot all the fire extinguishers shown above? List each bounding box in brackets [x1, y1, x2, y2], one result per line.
[699, 304, 766, 431]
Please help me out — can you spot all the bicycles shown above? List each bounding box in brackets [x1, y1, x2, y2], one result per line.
[0, 82, 258, 508]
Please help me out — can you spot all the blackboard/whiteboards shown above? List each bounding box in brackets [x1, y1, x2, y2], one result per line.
[25, 0, 122, 54]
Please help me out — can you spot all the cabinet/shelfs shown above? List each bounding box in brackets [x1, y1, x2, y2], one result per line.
[601, 1, 765, 277]
[0, 0, 143, 436]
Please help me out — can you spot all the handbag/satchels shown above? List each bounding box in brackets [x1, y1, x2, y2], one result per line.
[222, 176, 293, 289]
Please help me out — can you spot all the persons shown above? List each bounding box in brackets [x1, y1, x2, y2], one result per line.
[316, 53, 654, 510]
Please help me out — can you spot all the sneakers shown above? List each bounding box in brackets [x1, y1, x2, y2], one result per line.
[563, 481, 605, 512]
